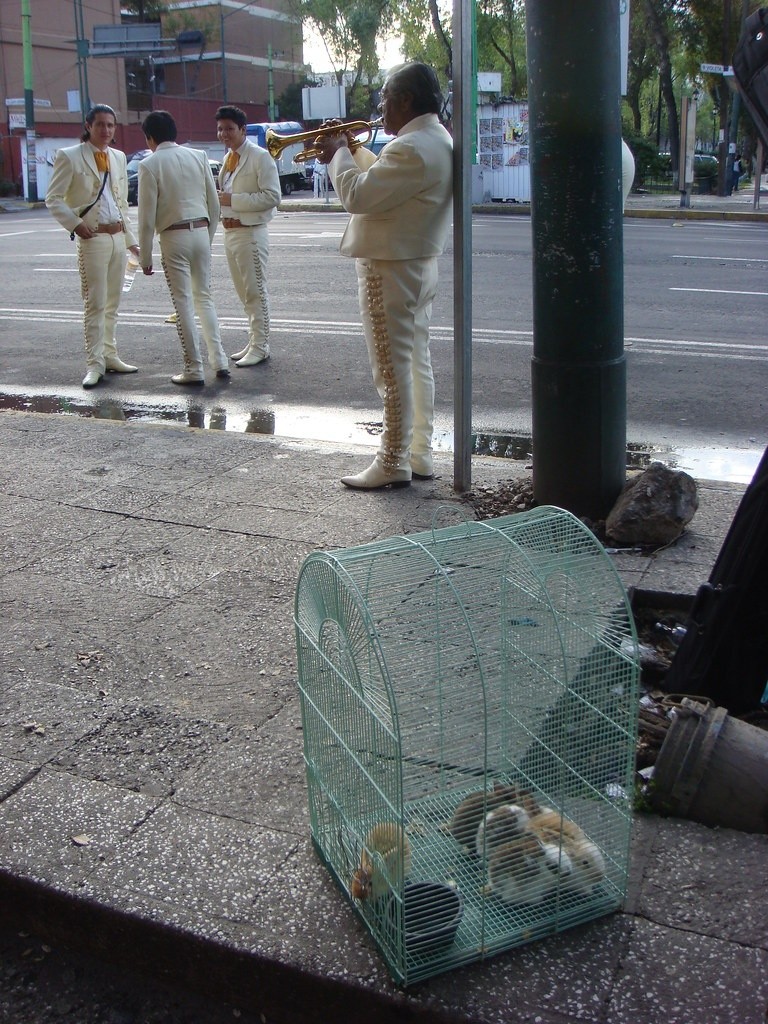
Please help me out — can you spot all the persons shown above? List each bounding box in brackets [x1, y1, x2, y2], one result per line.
[313, 156, 324, 199]
[215, 105, 281, 366]
[45, 104, 142, 387]
[664, 158, 672, 177]
[137, 111, 231, 382]
[744, 159, 752, 179]
[313, 64, 454, 492]
[734, 155, 744, 192]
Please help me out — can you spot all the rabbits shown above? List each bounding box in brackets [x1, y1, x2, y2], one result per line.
[352, 823, 411, 900]
[437, 779, 606, 904]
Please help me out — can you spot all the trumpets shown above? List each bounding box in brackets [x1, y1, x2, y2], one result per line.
[265, 116, 384, 163]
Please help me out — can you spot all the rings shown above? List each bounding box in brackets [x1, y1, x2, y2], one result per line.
[321, 151, 324, 154]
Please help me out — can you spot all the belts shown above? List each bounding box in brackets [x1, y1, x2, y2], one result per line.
[93, 222, 124, 234]
[165, 218, 210, 230]
[222, 217, 262, 229]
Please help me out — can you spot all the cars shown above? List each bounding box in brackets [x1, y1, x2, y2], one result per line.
[207, 159, 224, 192]
[356, 129, 398, 158]
[302, 157, 334, 192]
[124, 149, 154, 206]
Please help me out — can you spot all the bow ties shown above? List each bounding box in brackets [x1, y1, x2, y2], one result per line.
[94, 151, 109, 172]
[225, 151, 240, 172]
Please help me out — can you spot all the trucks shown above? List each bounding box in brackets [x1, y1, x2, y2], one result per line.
[225, 121, 306, 194]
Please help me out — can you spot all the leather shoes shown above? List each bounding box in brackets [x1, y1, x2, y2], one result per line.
[104, 358, 138, 373]
[229, 346, 250, 360]
[171, 373, 204, 386]
[341, 457, 412, 490]
[235, 354, 263, 366]
[215, 366, 231, 377]
[82, 371, 103, 389]
[411, 453, 434, 480]
